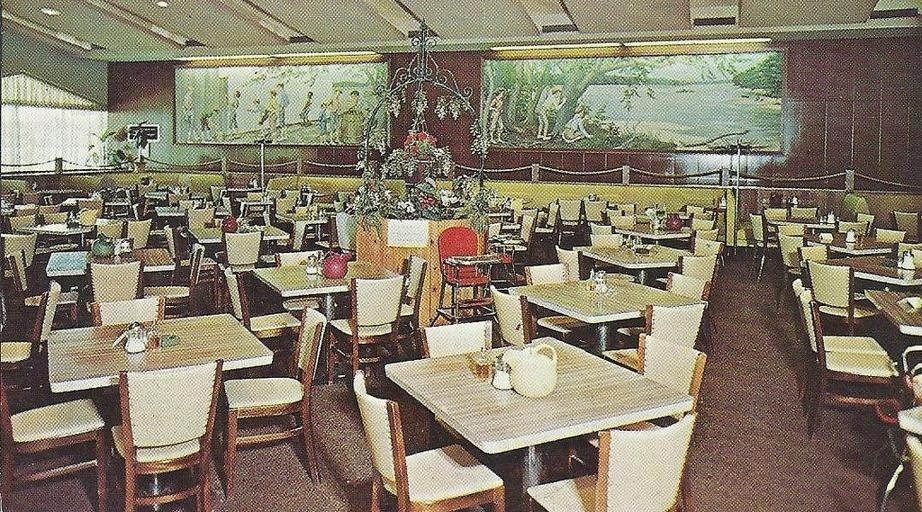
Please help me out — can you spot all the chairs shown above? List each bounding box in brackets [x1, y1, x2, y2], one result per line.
[0, 181, 922, 512]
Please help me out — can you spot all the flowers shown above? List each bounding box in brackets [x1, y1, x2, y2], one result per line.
[380, 128, 456, 182]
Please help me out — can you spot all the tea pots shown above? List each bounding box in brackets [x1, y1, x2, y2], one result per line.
[321, 251, 355, 279]
[219, 214, 238, 234]
[90, 234, 114, 258]
[502, 342, 559, 398]
[663, 212, 687, 232]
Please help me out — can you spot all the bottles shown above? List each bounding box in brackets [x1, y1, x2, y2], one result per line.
[819, 213, 839, 224]
[587, 271, 610, 293]
[119, 240, 133, 259]
[897, 250, 914, 269]
[305, 254, 317, 276]
[490, 352, 513, 390]
[123, 320, 164, 353]
[845, 229, 863, 244]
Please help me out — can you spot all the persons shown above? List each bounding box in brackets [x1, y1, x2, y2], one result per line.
[560, 107, 594, 143]
[536, 87, 566, 140]
[181, 83, 365, 145]
[488, 88, 506, 145]
